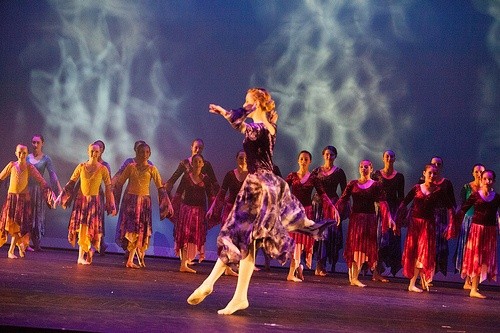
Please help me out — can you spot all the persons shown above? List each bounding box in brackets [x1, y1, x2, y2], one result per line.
[0, 134, 500, 298]
[186, 87, 337, 316]
